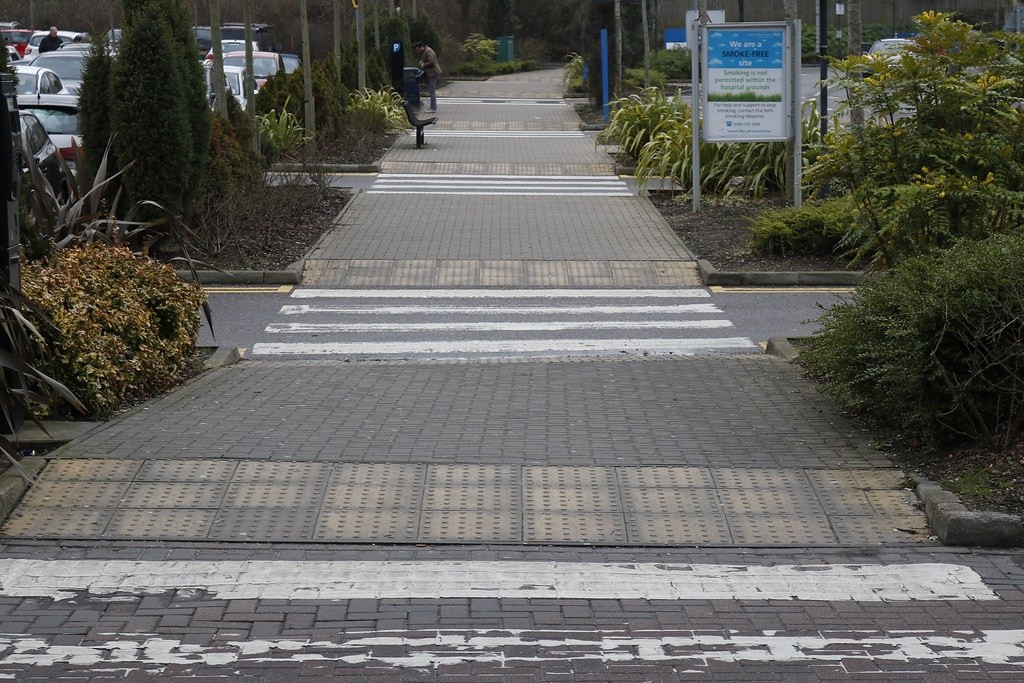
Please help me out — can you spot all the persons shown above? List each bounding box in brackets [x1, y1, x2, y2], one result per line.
[416, 42, 442, 113]
[38, 26, 63, 53]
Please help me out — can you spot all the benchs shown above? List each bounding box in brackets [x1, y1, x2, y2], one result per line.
[402, 100, 439, 149]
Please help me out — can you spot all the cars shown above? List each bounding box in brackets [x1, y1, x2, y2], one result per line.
[860, 38, 915, 79]
[0, 21, 300, 228]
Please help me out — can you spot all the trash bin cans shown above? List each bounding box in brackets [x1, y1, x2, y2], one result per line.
[403, 67, 420, 109]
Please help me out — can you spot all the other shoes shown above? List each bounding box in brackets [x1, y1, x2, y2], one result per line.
[424, 108, 437, 113]
